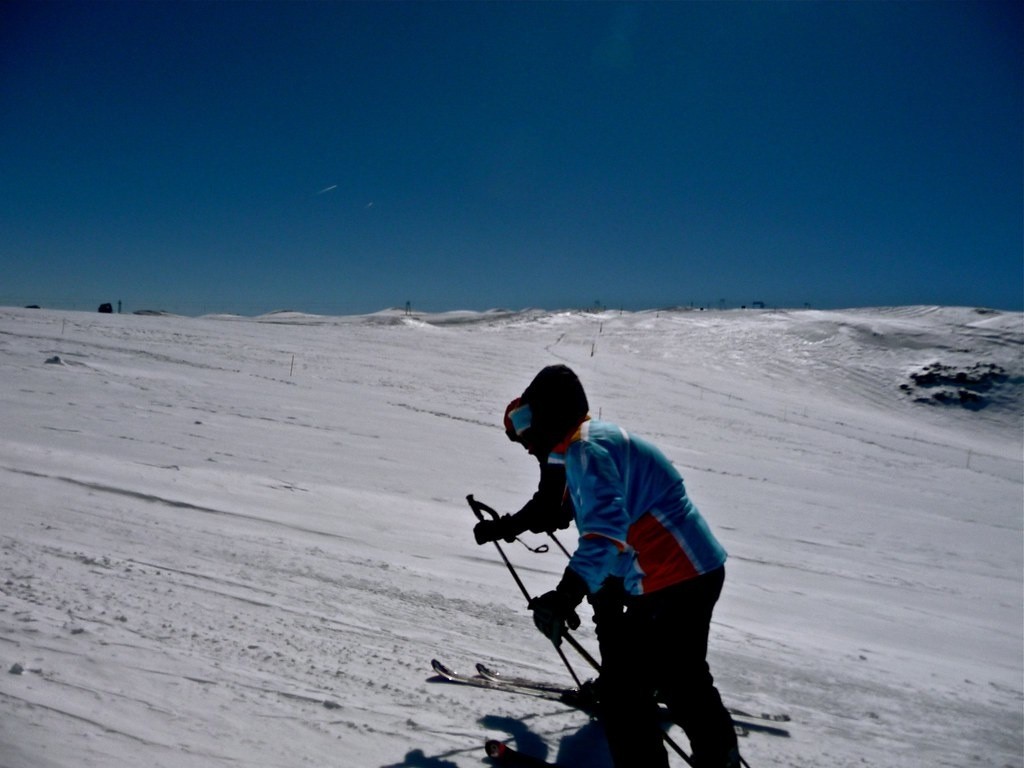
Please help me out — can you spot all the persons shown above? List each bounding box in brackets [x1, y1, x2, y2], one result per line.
[473, 395, 664, 719]
[508, 364, 740, 768]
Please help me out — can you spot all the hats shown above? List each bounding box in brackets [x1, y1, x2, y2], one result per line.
[504, 396, 525, 441]
[522, 364, 589, 466]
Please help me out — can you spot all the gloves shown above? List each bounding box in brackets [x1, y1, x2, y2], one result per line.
[473, 512, 515, 545]
[532, 566, 592, 647]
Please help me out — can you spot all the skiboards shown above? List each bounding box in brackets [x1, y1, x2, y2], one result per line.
[430, 658, 791, 736]
[485, 739, 568, 768]
